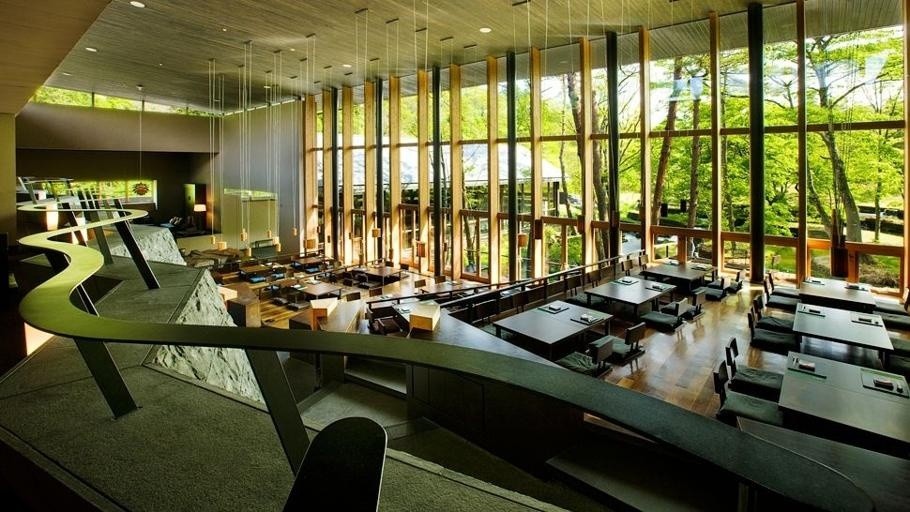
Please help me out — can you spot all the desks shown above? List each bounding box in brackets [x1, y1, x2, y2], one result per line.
[641, 260, 717, 294]
[799, 281, 877, 309]
[777, 350, 910, 451]
[493, 299, 613, 359]
[582, 276, 678, 321]
[792, 302, 895, 352]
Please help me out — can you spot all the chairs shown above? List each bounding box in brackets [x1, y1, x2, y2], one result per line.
[555, 340, 615, 380]
[883, 333, 909, 375]
[714, 336, 787, 433]
[690, 276, 732, 301]
[268, 262, 492, 327]
[746, 295, 801, 355]
[873, 289, 910, 331]
[588, 322, 648, 367]
[661, 290, 707, 320]
[640, 297, 688, 338]
[762, 269, 800, 311]
[707, 269, 746, 294]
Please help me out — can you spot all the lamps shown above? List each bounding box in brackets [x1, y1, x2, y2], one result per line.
[415, 12, 585, 258]
[207, 9, 383, 259]
[656, 11, 688, 218]
